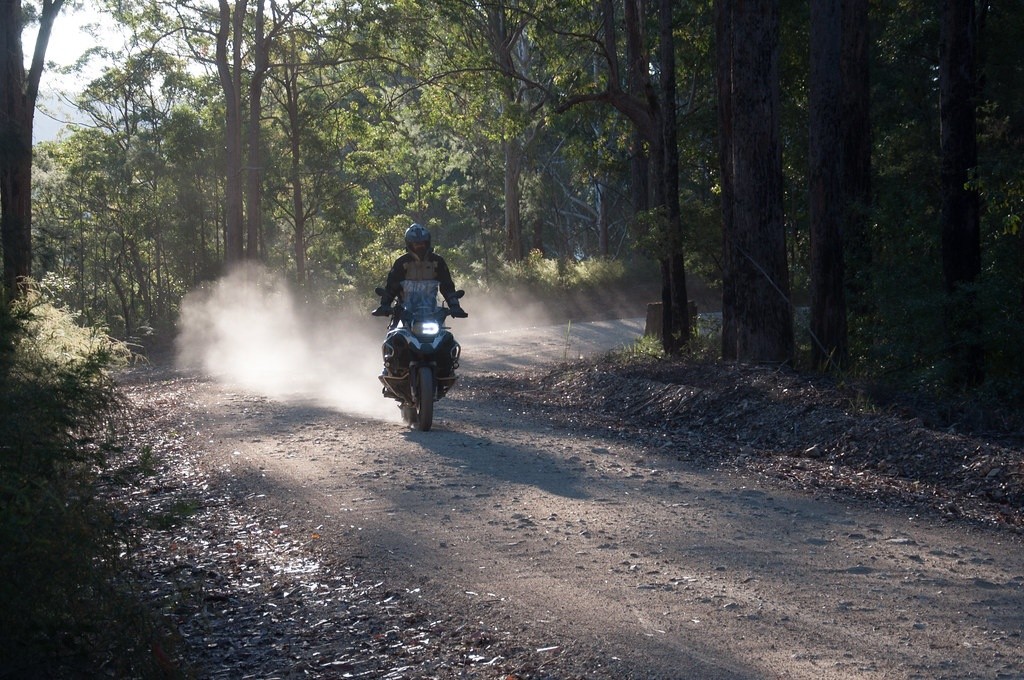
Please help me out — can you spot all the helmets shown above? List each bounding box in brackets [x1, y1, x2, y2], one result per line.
[404, 223, 431, 251]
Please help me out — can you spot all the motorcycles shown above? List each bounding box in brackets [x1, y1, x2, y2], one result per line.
[372, 286, 469, 434]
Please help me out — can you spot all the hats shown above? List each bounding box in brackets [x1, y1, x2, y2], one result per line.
[450, 307, 467, 320]
[371, 306, 391, 317]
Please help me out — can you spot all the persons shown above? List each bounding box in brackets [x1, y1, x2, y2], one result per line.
[371, 223, 468, 390]
